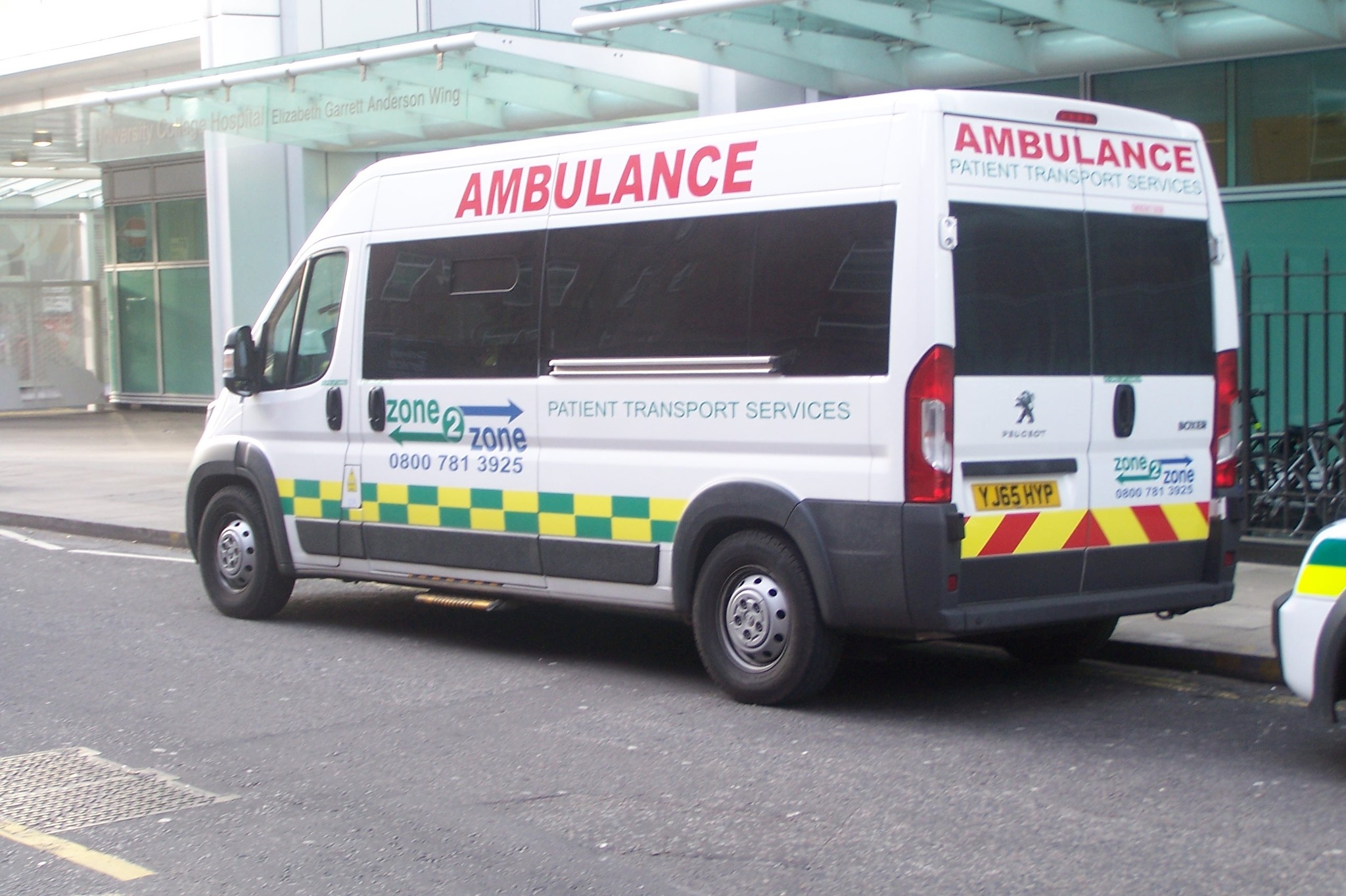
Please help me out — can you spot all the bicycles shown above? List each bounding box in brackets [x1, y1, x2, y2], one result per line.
[1225, 387, 1346, 539]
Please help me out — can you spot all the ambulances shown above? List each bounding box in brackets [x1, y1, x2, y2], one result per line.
[185, 85, 1243, 710]
[1270, 520, 1346, 729]
